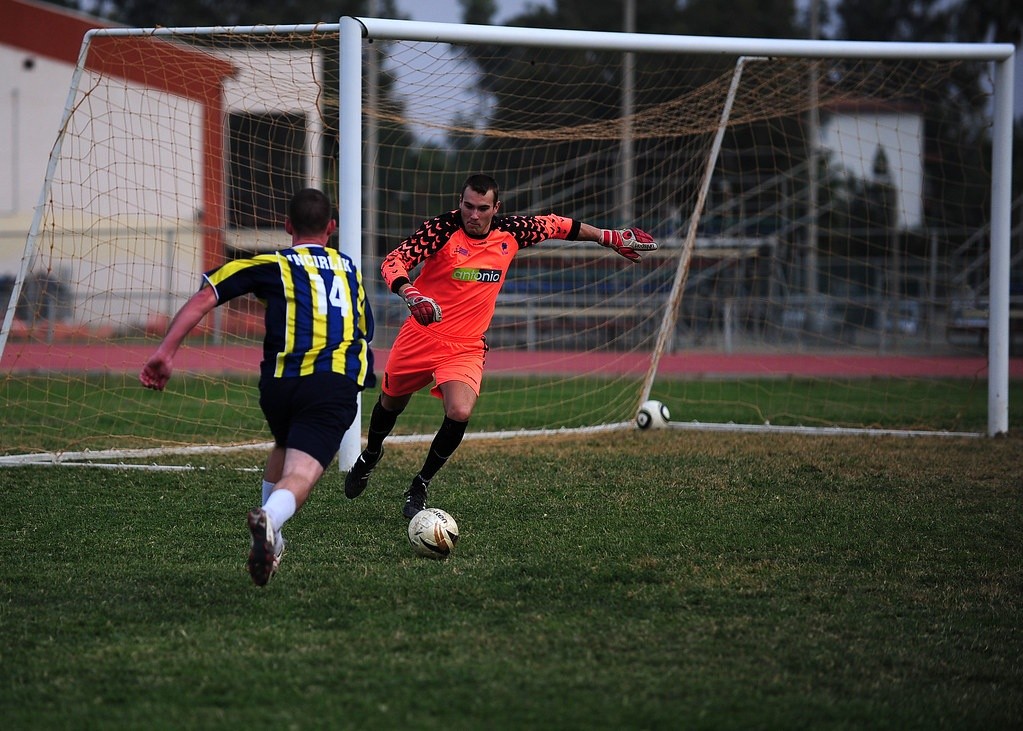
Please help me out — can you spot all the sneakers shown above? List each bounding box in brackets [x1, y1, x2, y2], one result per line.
[245, 509, 283, 589]
[345, 444, 385, 499]
[401, 474, 431, 518]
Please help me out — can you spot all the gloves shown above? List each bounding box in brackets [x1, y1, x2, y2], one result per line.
[599, 227, 658, 264]
[402, 287, 443, 326]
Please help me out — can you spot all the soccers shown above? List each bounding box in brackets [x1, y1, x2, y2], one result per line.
[406, 508, 458, 560]
[635, 400, 671, 432]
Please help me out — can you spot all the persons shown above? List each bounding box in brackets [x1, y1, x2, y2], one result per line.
[138, 188, 378, 585]
[343, 172, 657, 522]
